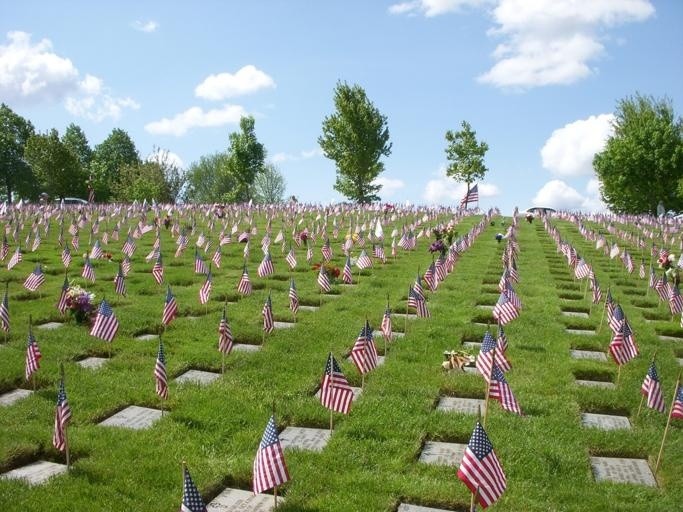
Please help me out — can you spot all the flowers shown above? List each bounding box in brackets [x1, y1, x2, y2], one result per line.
[495, 232, 503, 240]
[64, 285, 99, 316]
[657, 252, 675, 269]
[526, 213, 535, 221]
[428, 240, 448, 254]
[440, 227, 458, 241]
[311, 262, 340, 279]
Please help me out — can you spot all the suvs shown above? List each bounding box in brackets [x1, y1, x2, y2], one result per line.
[665, 207, 683, 224]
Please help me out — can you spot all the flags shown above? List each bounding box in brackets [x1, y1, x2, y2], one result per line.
[1, 199, 682, 511]
[461, 183, 478, 203]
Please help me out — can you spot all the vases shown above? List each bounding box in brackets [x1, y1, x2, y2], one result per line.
[75, 311, 85, 323]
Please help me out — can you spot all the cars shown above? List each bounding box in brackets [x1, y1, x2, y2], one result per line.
[515, 205, 557, 216]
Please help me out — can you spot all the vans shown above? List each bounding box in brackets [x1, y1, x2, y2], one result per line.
[52, 197, 97, 212]
